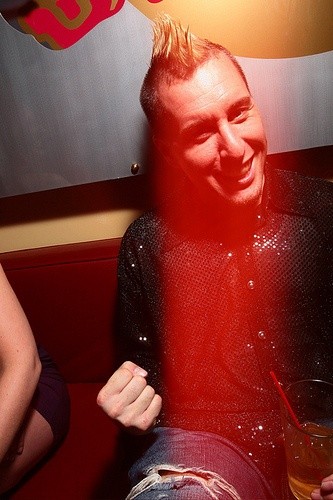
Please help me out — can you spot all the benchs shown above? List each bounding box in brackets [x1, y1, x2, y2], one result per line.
[0, 238, 129, 500]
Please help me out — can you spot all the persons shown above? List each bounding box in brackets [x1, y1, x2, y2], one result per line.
[0, 265, 70, 500]
[97, 21, 333, 500]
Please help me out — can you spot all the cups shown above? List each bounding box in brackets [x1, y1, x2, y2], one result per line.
[279, 379, 333, 500]
